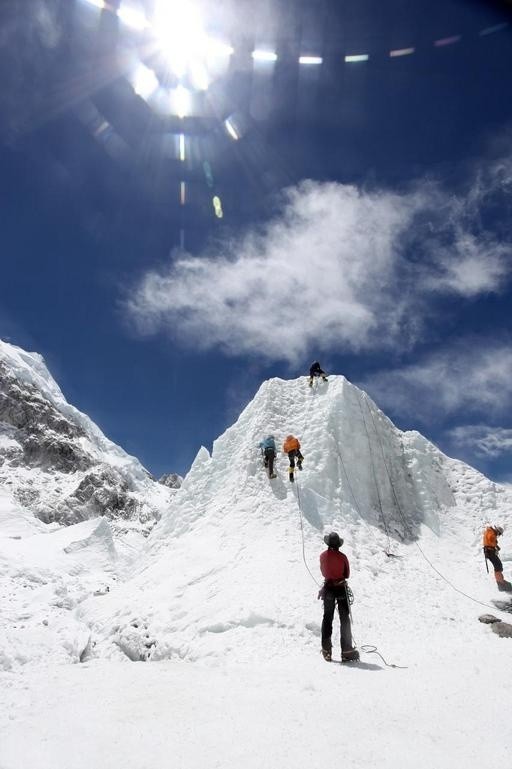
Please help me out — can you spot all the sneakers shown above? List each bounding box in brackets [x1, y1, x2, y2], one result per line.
[342, 649, 359, 660]
[322, 650, 331, 660]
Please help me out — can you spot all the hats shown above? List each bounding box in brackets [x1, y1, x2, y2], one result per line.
[494, 526, 504, 535]
[324, 532, 343, 548]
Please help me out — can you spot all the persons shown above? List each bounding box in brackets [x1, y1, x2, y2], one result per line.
[319, 532, 360, 661]
[283, 435, 304, 482]
[309, 360, 328, 385]
[482, 522, 511, 590]
[257, 434, 278, 478]
